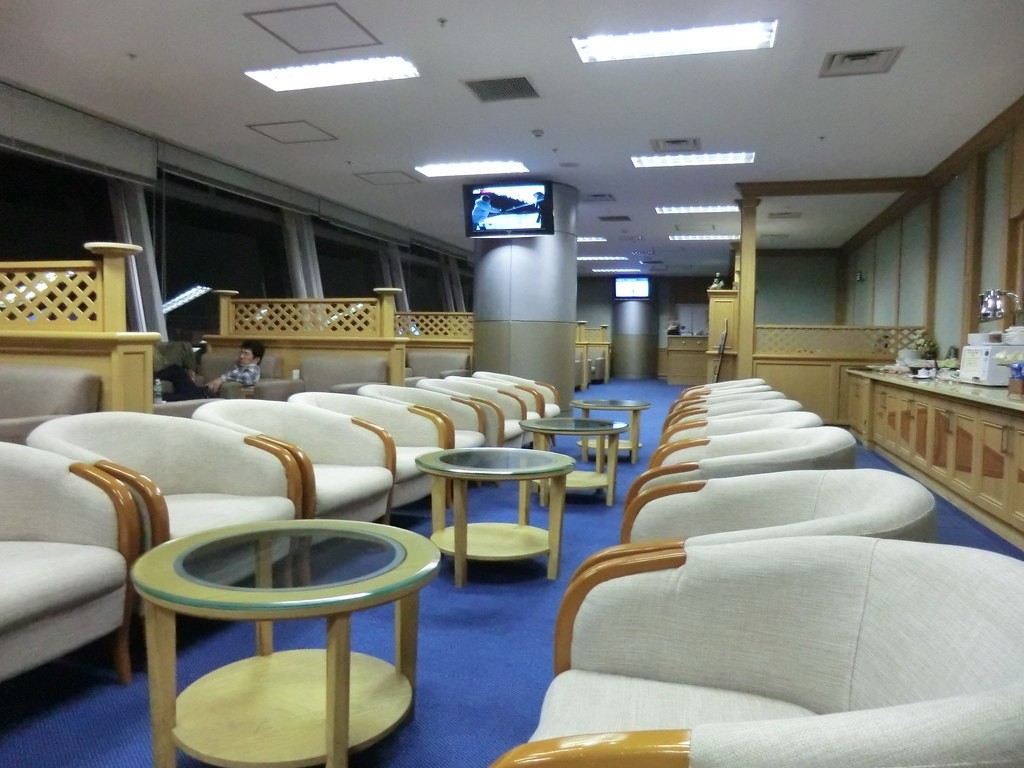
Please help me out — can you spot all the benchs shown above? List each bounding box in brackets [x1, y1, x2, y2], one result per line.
[0, 343, 607, 446]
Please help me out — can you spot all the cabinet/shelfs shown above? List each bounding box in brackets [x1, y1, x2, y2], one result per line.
[846, 372, 871, 441]
[871, 380, 1024, 533]
[666, 334, 708, 385]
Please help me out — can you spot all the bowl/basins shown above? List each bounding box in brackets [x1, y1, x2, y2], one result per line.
[909, 366, 932, 375]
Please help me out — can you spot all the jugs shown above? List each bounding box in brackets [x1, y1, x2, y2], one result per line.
[1004, 326, 1024, 345]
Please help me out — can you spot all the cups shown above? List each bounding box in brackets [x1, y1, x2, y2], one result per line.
[918, 370, 930, 376]
[988, 333, 1002, 343]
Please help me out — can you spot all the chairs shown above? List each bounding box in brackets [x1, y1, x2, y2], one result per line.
[488, 375, 1024, 768]
[0, 370, 559, 683]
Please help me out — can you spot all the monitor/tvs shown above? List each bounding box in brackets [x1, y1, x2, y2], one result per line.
[613, 276, 652, 301]
[463, 180, 555, 237]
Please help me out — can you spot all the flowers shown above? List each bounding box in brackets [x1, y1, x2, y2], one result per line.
[909, 329, 940, 360]
[993, 350, 1024, 379]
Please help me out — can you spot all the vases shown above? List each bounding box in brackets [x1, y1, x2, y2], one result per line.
[1007, 378, 1024, 397]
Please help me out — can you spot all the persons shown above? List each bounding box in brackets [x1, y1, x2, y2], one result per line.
[153, 339, 265, 402]
[532, 191, 544, 230]
[472, 194, 505, 230]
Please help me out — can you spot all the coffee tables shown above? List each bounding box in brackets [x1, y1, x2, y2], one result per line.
[517, 419, 629, 507]
[568, 399, 652, 464]
[412, 448, 576, 589]
[128, 517, 441, 767]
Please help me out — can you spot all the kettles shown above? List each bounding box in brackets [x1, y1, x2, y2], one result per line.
[977, 288, 1019, 321]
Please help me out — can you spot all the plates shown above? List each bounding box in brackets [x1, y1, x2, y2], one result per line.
[907, 374, 931, 378]
[984, 342, 1004, 345]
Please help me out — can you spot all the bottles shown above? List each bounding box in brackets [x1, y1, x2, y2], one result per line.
[153, 379, 162, 403]
